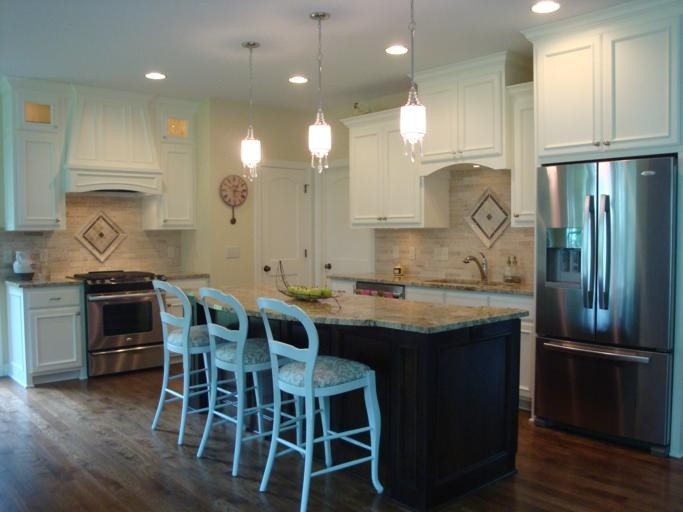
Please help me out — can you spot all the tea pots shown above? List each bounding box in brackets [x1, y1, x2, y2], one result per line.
[11, 250, 32, 274]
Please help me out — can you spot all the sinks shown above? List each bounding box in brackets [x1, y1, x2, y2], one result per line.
[422, 278, 502, 286]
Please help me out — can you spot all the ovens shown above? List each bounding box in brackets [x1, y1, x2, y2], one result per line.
[83, 291, 166, 378]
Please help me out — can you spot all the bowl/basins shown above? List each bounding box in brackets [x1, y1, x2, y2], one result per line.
[13, 272, 35, 282]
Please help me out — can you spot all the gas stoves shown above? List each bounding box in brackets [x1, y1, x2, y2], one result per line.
[64, 269, 166, 291]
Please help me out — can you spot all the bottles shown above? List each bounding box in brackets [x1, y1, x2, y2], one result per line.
[502, 254, 521, 284]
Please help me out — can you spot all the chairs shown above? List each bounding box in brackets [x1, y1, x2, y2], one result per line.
[255, 294, 388, 512]
[194, 285, 303, 476]
[146, 280, 213, 446]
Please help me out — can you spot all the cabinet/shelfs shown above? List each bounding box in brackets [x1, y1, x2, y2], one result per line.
[11, 80, 68, 235]
[340, 113, 450, 229]
[163, 276, 212, 367]
[6, 281, 89, 389]
[143, 97, 197, 235]
[407, 50, 509, 178]
[326, 275, 534, 411]
[65, 83, 154, 165]
[522, 0, 683, 168]
[500, 82, 537, 229]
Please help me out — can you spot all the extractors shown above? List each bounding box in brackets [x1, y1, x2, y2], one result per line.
[61, 171, 163, 199]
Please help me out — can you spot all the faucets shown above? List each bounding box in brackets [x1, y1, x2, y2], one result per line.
[463, 252, 489, 285]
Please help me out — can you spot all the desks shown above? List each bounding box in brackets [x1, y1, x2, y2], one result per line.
[189, 282, 529, 512]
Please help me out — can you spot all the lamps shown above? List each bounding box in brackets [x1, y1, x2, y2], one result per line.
[237, 41, 263, 180]
[396, 0, 427, 163]
[306, 10, 333, 176]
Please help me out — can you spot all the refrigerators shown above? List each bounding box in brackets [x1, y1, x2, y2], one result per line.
[532, 152, 677, 452]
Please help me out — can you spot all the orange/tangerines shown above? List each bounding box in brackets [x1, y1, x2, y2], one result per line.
[288, 285, 333, 301]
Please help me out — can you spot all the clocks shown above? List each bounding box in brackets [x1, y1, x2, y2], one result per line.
[218, 175, 248, 207]
